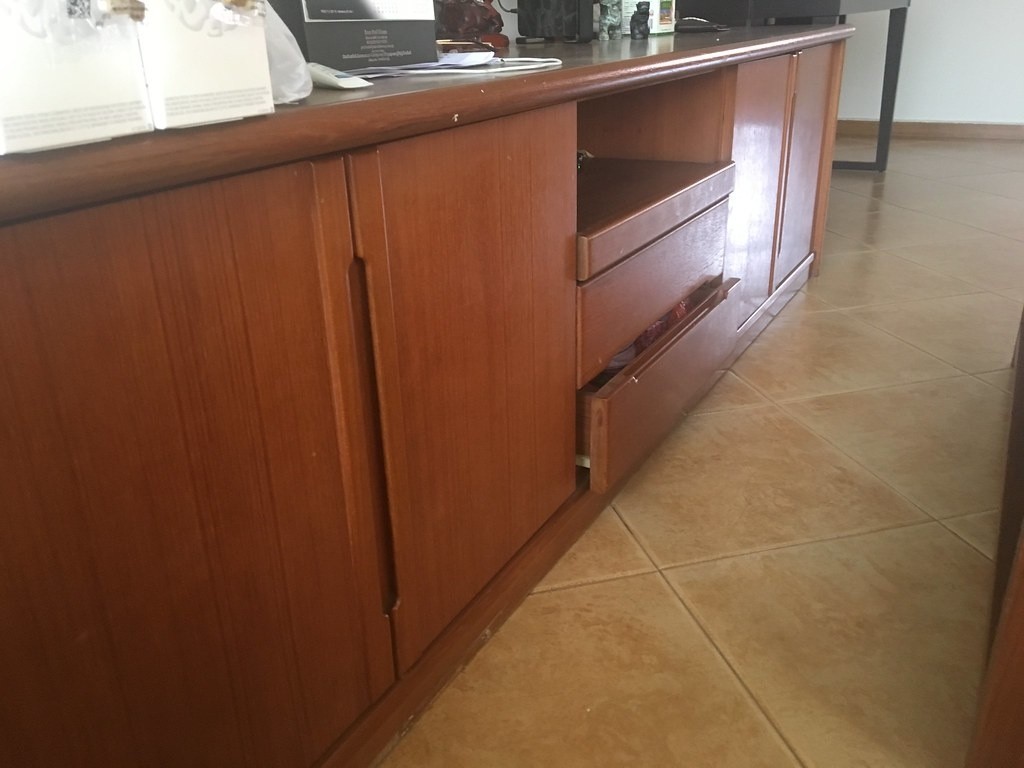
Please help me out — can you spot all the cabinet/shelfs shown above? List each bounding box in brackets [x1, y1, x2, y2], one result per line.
[0, 24, 859, 768]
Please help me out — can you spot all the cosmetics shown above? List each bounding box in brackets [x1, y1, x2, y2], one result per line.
[304, 60, 374, 90]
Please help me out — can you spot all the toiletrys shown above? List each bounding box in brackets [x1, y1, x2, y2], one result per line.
[305, 61, 375, 90]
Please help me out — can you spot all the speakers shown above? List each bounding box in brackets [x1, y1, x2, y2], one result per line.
[517, 0, 592, 42]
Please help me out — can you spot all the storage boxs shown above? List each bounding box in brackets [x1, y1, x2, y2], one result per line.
[282, 21, 439, 73]
[268, 0, 436, 22]
[594, 0, 676, 37]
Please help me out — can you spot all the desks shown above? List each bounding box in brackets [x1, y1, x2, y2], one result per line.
[676, 0, 911, 170]
